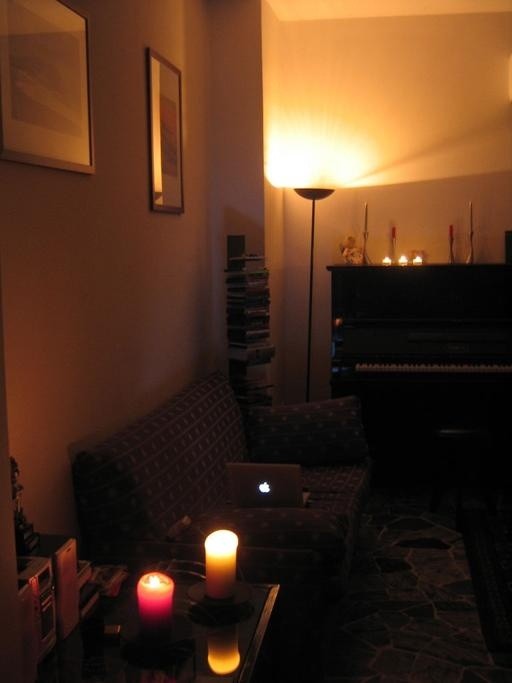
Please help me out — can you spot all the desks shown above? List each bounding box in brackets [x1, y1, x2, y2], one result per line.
[46, 572, 281, 682]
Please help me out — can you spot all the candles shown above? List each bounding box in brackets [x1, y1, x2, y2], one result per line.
[137, 570, 175, 632]
[203, 527, 238, 597]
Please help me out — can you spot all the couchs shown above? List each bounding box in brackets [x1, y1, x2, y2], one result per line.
[71, 366, 381, 655]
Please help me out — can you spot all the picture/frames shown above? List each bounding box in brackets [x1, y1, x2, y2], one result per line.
[145, 44, 185, 217]
[0, 0, 94, 173]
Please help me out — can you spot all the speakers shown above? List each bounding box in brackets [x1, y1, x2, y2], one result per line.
[18, 578, 37, 683]
[25, 535, 81, 642]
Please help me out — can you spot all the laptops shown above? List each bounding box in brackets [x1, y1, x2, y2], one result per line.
[225, 461, 310, 508]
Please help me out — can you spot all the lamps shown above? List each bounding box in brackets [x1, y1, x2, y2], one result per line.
[292, 187, 336, 405]
